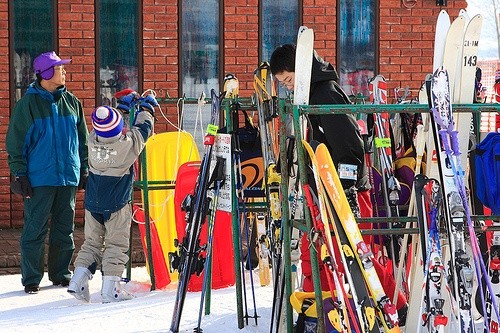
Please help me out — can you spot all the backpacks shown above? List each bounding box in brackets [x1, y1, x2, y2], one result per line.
[475, 132, 500, 218]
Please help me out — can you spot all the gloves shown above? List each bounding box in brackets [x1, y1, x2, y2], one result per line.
[140, 94, 158, 113]
[116, 91, 141, 114]
[77, 176, 89, 190]
[345, 185, 361, 224]
[10, 176, 33, 199]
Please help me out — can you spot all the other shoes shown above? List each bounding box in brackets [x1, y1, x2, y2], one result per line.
[24, 283, 40, 293]
[292, 313, 319, 333]
[385, 303, 408, 329]
[53, 275, 71, 286]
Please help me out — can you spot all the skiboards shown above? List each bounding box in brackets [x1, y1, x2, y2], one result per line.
[169, 8, 500, 333]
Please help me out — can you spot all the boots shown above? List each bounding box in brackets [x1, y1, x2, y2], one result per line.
[102, 275, 133, 303]
[67, 266, 93, 304]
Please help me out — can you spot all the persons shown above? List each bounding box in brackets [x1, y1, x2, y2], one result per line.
[270, 42, 407, 333]
[5, 51, 90, 294]
[67, 91, 158, 303]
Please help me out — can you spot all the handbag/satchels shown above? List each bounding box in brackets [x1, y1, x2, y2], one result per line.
[215, 108, 264, 193]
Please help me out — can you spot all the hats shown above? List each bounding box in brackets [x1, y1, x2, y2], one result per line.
[90, 105, 124, 137]
[32, 51, 73, 79]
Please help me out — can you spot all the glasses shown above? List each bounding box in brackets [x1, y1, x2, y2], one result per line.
[278, 80, 294, 89]
